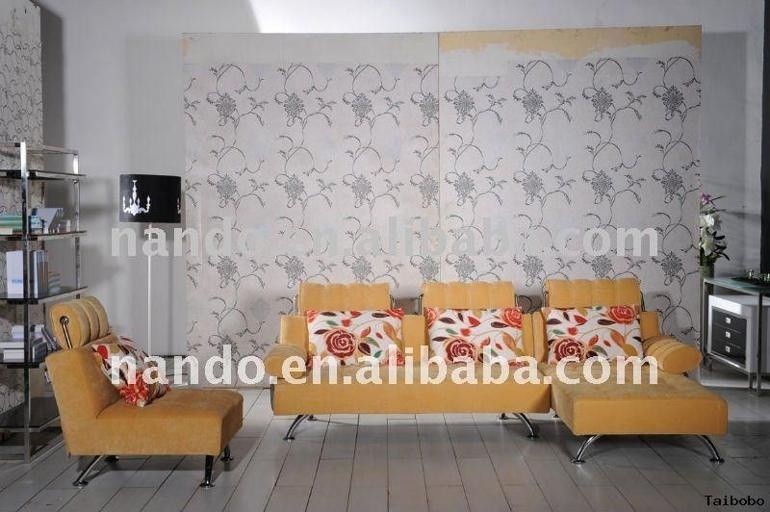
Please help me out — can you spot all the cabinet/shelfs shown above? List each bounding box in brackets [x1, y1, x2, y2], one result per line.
[0, 142, 87, 464]
[702, 276, 770, 395]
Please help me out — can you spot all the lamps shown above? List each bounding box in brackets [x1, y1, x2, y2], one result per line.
[119, 176, 180, 357]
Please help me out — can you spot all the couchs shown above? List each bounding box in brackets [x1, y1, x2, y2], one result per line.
[45, 296, 244, 489]
[263, 279, 551, 440]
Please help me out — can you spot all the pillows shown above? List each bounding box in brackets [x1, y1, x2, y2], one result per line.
[541, 305, 648, 365]
[423, 306, 529, 367]
[90, 334, 171, 408]
[305, 308, 405, 369]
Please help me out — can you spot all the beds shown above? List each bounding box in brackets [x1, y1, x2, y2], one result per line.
[532, 279, 728, 466]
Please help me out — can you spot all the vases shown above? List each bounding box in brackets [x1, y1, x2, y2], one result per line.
[700, 264, 713, 352]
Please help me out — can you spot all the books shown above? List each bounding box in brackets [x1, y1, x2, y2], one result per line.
[0, 208, 75, 234]
[6, 249, 62, 299]
[0, 323, 56, 362]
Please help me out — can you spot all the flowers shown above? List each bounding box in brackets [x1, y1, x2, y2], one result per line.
[700, 192, 730, 265]
